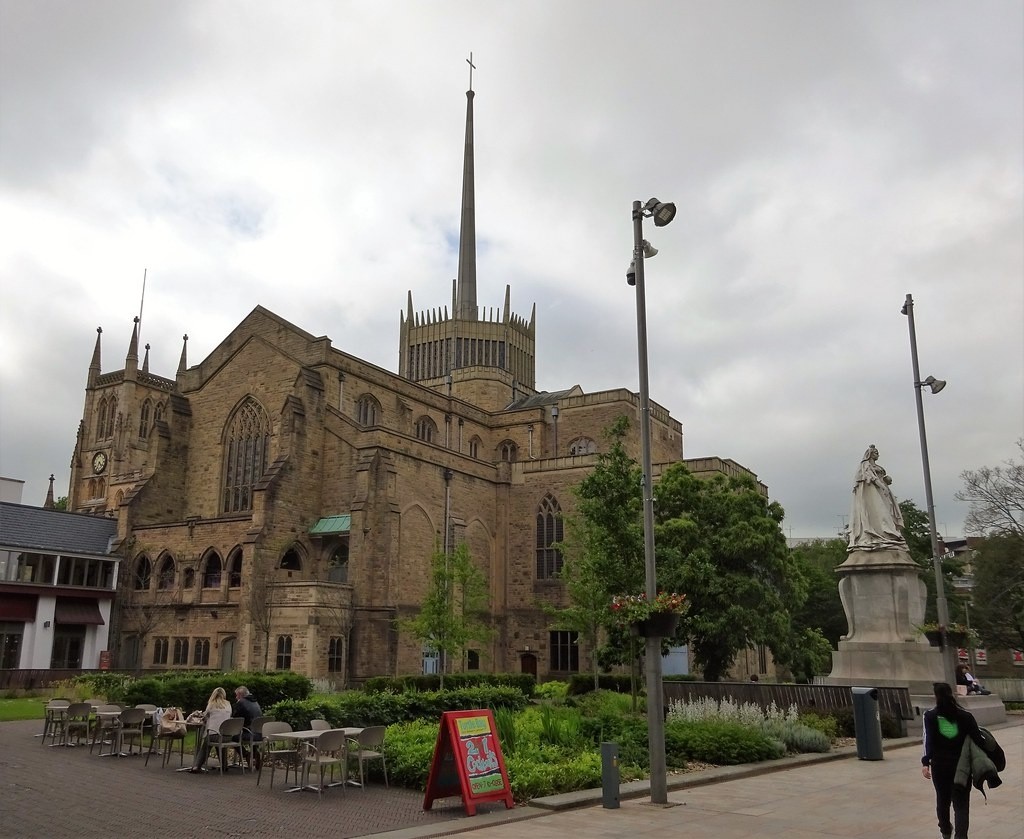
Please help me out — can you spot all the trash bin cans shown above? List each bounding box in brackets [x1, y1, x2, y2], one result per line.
[850, 686, 885, 761]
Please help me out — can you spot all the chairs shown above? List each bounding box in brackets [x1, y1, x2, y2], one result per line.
[345, 725, 389, 793]
[242, 716, 276, 773]
[256, 721, 299, 789]
[300, 729, 347, 800]
[144, 706, 186, 769]
[40, 697, 159, 758]
[205, 717, 245, 776]
[307, 719, 334, 785]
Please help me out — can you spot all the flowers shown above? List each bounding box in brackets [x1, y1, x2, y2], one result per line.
[612, 591, 687, 632]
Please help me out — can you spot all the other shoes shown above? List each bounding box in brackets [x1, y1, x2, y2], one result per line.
[189, 766, 201, 773]
[969, 690, 991, 695]
[943, 824, 953, 838]
[219, 766, 228, 771]
[245, 752, 254, 766]
[255, 752, 260, 770]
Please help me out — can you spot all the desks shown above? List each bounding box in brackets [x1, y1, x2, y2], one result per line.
[170, 718, 206, 759]
[270, 726, 365, 794]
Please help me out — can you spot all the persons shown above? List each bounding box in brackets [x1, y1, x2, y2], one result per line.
[921, 683, 1006, 839]
[750, 674, 758, 684]
[190, 688, 232, 772]
[956, 663, 991, 695]
[232, 686, 262, 770]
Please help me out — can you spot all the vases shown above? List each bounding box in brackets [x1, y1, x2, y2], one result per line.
[638, 611, 682, 638]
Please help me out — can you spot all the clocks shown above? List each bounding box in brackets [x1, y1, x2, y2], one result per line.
[92, 451, 107, 474]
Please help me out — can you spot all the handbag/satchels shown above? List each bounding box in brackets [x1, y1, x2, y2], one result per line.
[160, 708, 187, 737]
[187, 710, 204, 723]
